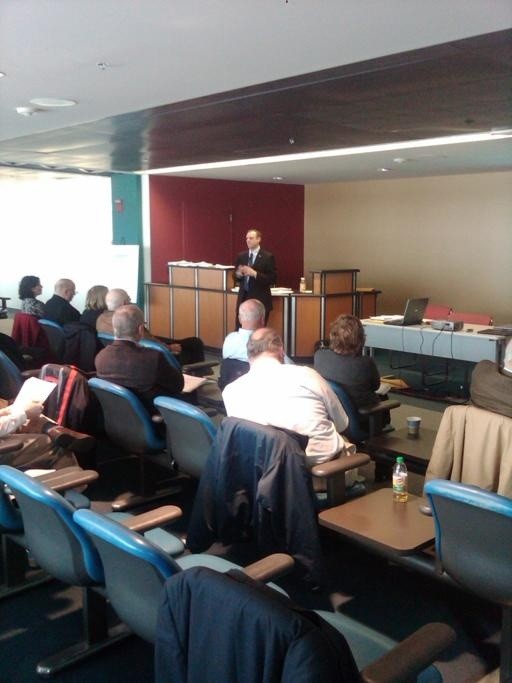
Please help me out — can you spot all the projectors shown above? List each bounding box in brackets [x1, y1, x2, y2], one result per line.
[432, 319, 464, 331]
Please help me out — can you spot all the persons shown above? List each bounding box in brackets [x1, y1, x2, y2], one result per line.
[0, 402, 114, 500]
[220, 326, 368, 495]
[222, 299, 293, 362]
[95, 304, 198, 441]
[18, 276, 205, 366]
[468, 335, 512, 417]
[229, 226, 277, 332]
[311, 313, 395, 432]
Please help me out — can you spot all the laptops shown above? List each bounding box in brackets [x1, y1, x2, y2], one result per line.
[383, 298, 429, 326]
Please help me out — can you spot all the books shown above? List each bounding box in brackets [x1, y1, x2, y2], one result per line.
[369, 313, 404, 321]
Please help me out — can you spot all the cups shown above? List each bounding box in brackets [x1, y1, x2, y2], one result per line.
[406, 416, 421, 435]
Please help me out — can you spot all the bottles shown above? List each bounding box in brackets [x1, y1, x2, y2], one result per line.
[391, 457, 408, 501]
[299, 277, 306, 292]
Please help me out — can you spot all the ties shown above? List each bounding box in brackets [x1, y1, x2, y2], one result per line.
[248, 253, 253, 269]
